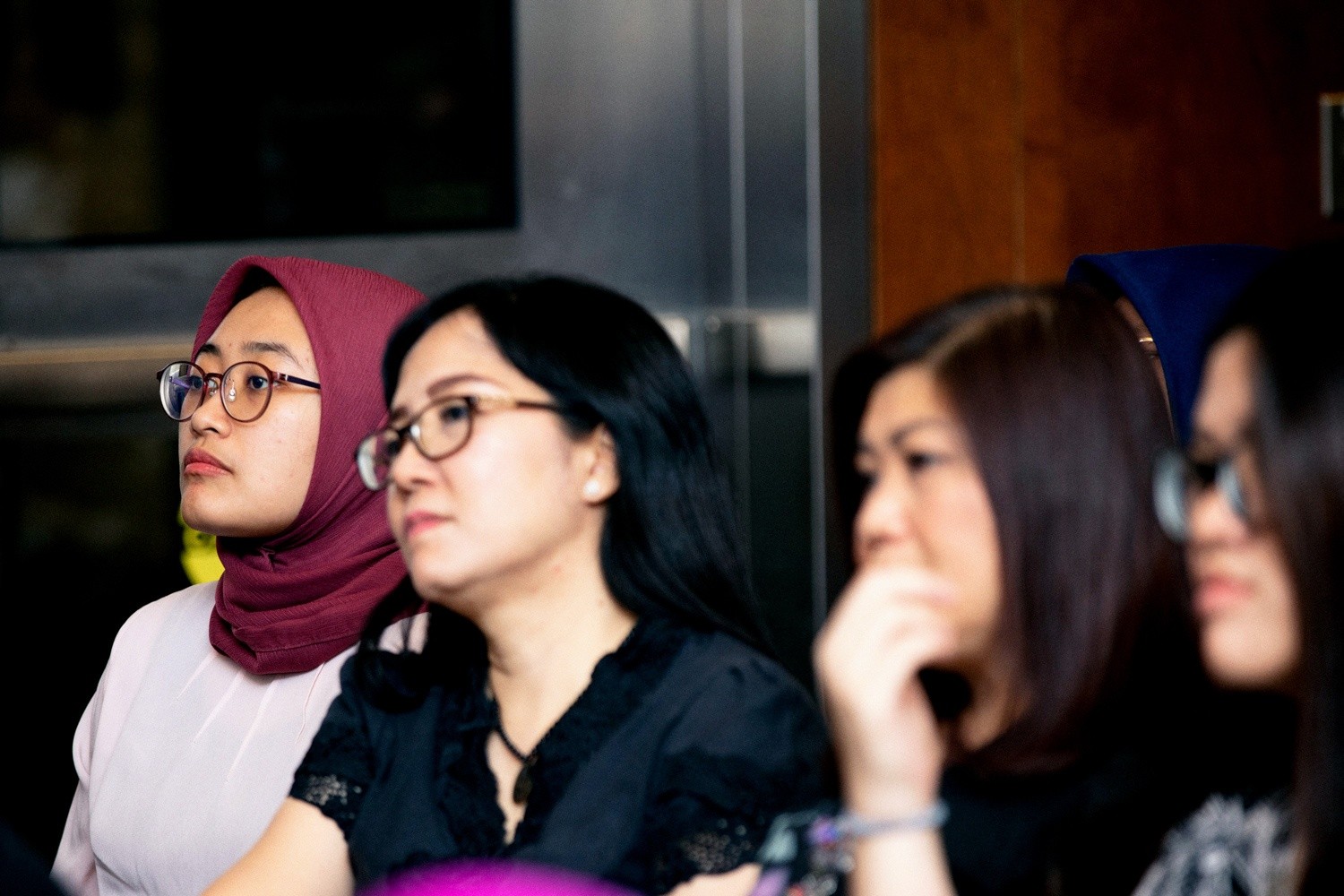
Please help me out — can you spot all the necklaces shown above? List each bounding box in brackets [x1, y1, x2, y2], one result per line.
[490, 712, 542, 806]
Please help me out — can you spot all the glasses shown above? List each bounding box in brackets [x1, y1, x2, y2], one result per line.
[1148, 419, 1273, 541]
[354, 394, 562, 490]
[156, 361, 321, 423]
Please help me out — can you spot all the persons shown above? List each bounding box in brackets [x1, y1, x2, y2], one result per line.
[667, 274, 1179, 895]
[199, 273, 834, 896]
[807, 274, 1343, 895]
[45, 252, 460, 896]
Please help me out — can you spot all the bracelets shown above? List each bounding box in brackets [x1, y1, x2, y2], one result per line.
[809, 804, 950, 873]
